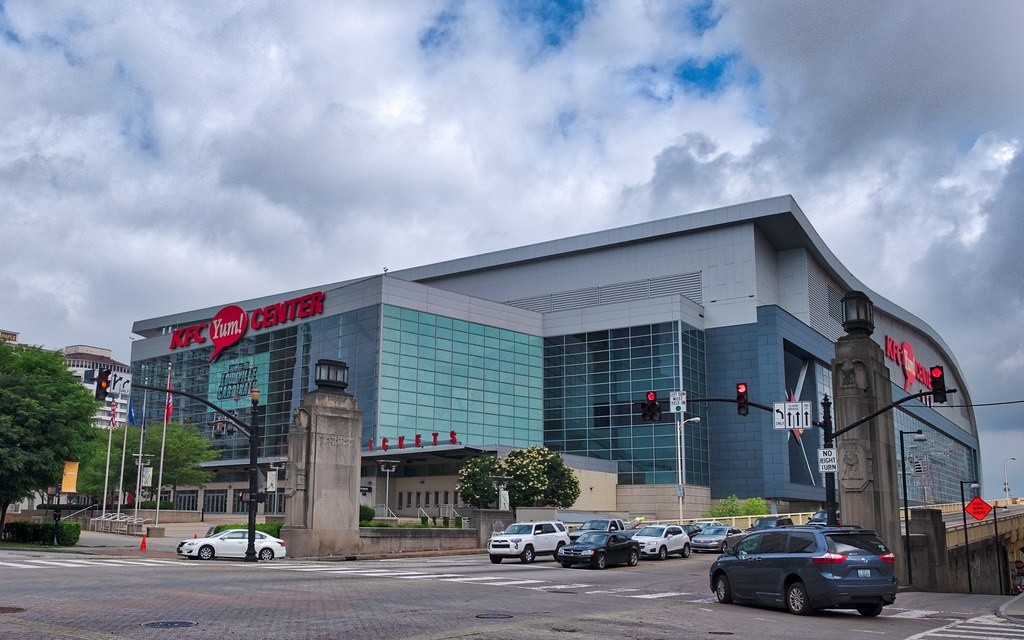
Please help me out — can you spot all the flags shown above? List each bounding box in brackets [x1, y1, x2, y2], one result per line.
[166, 377, 173, 425]
[109, 397, 116, 427]
[129, 399, 136, 426]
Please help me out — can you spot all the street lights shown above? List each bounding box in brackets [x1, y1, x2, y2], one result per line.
[244, 386, 265, 563]
[959, 480, 980, 593]
[900, 429, 927, 587]
[992, 505, 1011, 597]
[677, 417, 700, 525]
[1003, 456, 1015, 507]
[379, 459, 400, 517]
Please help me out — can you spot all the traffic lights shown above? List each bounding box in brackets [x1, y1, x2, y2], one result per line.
[929, 365, 947, 404]
[94, 366, 113, 401]
[48, 486, 57, 497]
[737, 382, 749, 416]
[640, 391, 661, 422]
[242, 493, 250, 503]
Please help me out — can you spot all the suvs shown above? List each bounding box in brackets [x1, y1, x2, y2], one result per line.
[708, 526, 897, 616]
[487, 521, 571, 564]
[745, 517, 795, 538]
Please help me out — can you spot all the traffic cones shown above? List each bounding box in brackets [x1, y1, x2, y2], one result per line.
[140, 534, 151, 552]
[194, 534, 198, 539]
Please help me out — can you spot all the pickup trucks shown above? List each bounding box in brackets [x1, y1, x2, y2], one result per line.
[569, 518, 642, 543]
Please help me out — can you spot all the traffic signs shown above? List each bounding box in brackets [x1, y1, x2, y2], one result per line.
[773, 401, 786, 430]
[112, 373, 134, 395]
[785, 402, 803, 429]
[802, 401, 813, 430]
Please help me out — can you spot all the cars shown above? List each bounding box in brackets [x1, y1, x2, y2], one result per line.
[631, 524, 691, 562]
[803, 511, 840, 529]
[691, 526, 748, 554]
[693, 521, 725, 533]
[678, 524, 702, 544]
[557, 530, 642, 570]
[1011, 570, 1024, 593]
[176, 528, 288, 561]
